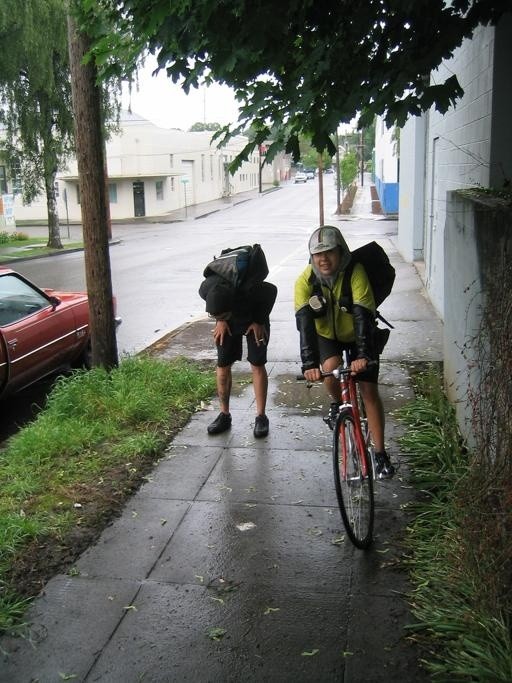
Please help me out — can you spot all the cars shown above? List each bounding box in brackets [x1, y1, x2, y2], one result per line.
[0, 261, 123, 410]
[292, 164, 336, 184]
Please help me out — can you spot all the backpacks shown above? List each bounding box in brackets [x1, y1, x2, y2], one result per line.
[201, 242, 270, 291]
[349, 239, 398, 312]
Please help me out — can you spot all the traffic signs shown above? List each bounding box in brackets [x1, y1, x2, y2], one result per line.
[180, 180, 190, 184]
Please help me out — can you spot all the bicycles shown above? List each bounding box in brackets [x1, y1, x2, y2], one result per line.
[292, 348, 379, 550]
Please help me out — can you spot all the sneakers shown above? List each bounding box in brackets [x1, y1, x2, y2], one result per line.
[254, 414, 269, 439]
[207, 412, 232, 435]
[373, 451, 396, 480]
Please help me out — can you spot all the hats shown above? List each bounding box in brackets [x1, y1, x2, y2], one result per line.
[308, 226, 342, 256]
[205, 284, 233, 313]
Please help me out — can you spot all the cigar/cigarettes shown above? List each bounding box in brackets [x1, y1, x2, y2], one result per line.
[258, 338, 264, 341]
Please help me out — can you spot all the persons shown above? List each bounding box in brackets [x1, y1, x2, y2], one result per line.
[198, 270, 279, 439]
[293, 224, 397, 480]
